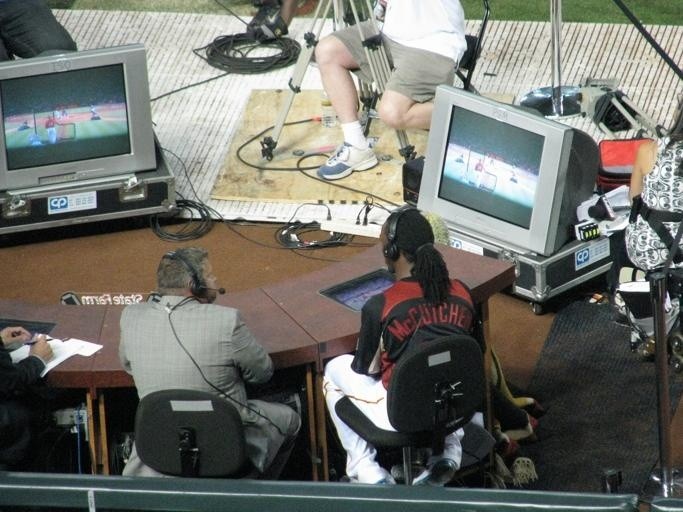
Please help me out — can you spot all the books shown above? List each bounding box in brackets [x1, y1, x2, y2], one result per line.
[9, 333, 76, 382]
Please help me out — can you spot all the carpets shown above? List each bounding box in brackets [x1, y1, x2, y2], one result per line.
[492, 296, 682, 512]
[211, 89, 517, 205]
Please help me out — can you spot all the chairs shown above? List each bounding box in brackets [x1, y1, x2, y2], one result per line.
[629, 194, 682, 373]
[133, 387, 244, 482]
[359, 0, 490, 138]
[334, 335, 485, 486]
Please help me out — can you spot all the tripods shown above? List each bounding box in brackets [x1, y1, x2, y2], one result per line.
[260, 0, 418, 164]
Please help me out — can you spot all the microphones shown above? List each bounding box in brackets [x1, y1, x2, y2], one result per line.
[200, 287, 225, 294]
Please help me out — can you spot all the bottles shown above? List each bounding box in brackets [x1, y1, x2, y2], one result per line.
[321, 91, 337, 127]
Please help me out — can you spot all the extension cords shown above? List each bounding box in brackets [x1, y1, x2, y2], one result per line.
[320, 217, 382, 239]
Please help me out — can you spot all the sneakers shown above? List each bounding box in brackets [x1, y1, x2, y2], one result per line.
[317, 142, 377, 180]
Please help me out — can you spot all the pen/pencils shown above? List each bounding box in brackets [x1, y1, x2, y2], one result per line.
[24, 339, 53, 345]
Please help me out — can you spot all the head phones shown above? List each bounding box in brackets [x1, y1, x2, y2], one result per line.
[164, 250, 207, 299]
[382, 206, 421, 259]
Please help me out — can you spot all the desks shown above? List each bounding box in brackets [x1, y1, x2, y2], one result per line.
[0, 299, 106, 474]
[95, 288, 321, 479]
[260, 243, 514, 482]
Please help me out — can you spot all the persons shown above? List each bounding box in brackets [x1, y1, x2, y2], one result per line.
[313, 0, 469, 181]
[118, 244, 301, 479]
[0, 325, 64, 474]
[603, 88, 683, 353]
[321, 204, 493, 485]
[3, 86, 125, 155]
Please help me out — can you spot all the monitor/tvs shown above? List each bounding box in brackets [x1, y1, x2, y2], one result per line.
[415, 83, 601, 255]
[0, 43, 157, 195]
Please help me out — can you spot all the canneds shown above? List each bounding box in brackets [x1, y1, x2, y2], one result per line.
[601, 466, 623, 495]
